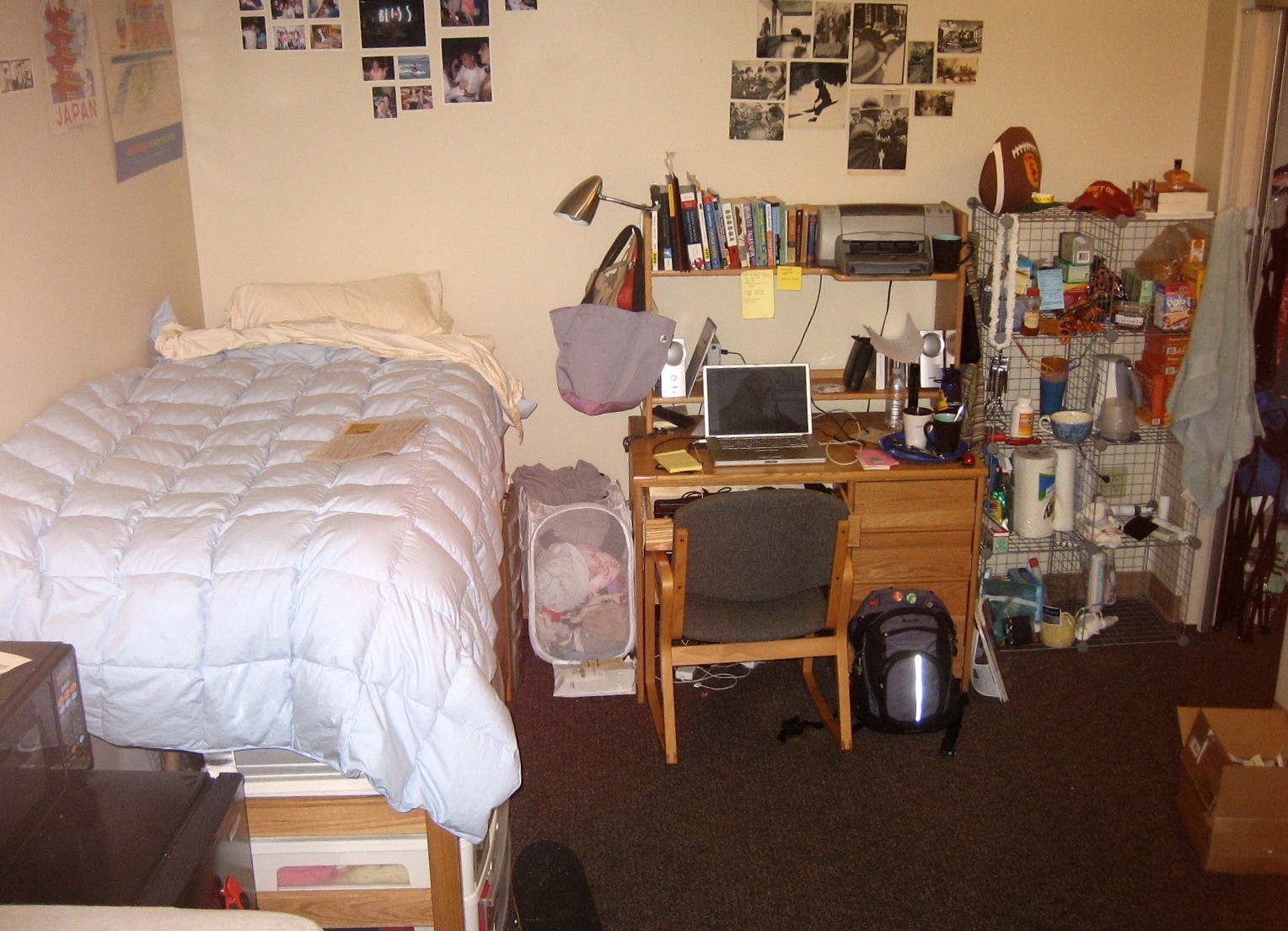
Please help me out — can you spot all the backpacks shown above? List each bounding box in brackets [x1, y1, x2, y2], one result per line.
[849, 586, 962, 758]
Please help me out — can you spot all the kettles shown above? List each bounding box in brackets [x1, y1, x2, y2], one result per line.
[1086, 353, 1142, 431]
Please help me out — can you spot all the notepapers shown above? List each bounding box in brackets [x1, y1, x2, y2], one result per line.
[652, 449, 702, 473]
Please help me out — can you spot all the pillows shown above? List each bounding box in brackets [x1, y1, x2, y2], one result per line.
[225, 270, 453, 338]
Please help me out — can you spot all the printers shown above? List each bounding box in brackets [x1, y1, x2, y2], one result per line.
[816, 204, 955, 276]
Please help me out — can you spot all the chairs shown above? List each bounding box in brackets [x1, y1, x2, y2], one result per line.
[644, 488, 854, 765]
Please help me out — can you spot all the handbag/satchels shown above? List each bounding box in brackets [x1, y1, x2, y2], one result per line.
[550, 226, 676, 418]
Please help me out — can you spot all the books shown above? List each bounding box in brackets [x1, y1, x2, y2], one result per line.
[856, 453, 891, 471]
[648, 176, 818, 270]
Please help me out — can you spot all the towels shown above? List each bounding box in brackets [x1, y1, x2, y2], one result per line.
[1165, 206, 1266, 521]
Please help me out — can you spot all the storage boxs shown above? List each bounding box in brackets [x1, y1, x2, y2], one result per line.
[1173, 705, 1288, 878]
[975, 222, 1213, 427]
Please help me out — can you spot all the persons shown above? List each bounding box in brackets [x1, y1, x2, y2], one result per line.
[729, 1, 908, 170]
[239, 0, 538, 119]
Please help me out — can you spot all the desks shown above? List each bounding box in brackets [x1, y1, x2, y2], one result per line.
[629, 411, 989, 706]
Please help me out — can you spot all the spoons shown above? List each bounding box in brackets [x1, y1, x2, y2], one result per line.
[885, 436, 945, 459]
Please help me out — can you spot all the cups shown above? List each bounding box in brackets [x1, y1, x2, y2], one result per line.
[902, 406, 933, 449]
[923, 413, 964, 454]
[932, 234, 974, 273]
[1038, 410, 1094, 443]
[1040, 357, 1069, 420]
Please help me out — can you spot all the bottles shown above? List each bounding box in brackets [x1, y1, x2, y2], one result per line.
[1126, 179, 1157, 211]
[884, 368, 906, 431]
[939, 364, 962, 412]
[1010, 396, 1035, 438]
[1163, 158, 1191, 190]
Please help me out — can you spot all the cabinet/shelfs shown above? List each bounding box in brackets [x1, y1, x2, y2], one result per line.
[636, 202, 971, 437]
[966, 195, 1217, 654]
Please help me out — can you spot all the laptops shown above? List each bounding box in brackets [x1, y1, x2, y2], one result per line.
[703, 363, 826, 466]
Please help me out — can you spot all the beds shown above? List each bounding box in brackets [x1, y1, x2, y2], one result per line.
[0, 299, 513, 931]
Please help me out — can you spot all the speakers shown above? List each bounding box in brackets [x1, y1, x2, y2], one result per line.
[654, 338, 686, 397]
[910, 329, 944, 388]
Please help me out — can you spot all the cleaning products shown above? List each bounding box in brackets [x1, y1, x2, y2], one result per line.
[1027, 557, 1045, 634]
[1086, 548, 1117, 614]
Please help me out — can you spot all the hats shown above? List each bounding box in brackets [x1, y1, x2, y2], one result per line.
[1067, 180, 1136, 220]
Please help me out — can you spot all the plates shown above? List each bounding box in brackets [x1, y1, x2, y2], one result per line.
[878, 432, 969, 463]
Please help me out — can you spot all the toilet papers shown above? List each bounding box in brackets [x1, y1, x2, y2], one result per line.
[1011, 443, 1077, 539]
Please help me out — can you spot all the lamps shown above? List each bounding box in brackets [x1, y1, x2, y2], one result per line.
[553, 175, 663, 227]
